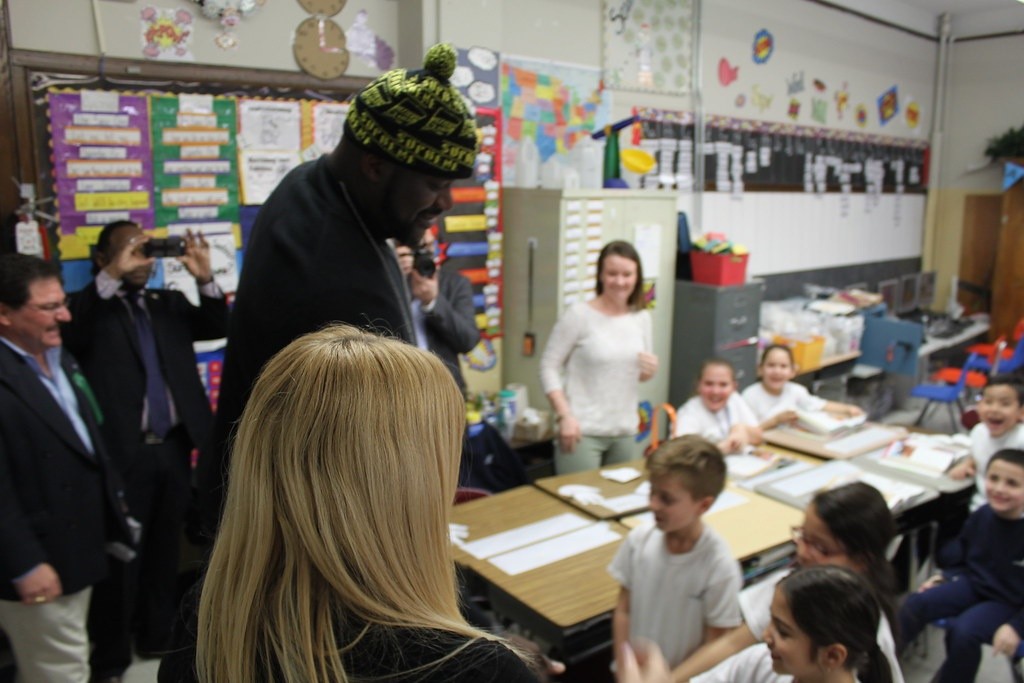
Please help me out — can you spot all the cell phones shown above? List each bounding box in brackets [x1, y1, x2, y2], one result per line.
[146, 237, 185, 258]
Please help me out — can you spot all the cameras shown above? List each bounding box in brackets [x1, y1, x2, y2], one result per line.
[408, 245, 436, 277]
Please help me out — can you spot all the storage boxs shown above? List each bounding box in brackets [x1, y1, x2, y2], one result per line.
[690, 232, 761, 287]
[757, 304, 863, 376]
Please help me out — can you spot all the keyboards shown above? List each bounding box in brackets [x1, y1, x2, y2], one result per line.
[934, 327, 964, 340]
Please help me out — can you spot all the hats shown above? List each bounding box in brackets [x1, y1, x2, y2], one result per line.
[343, 43, 478, 178]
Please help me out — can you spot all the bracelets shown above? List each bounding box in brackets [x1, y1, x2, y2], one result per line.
[557, 413, 569, 422]
[196, 277, 214, 286]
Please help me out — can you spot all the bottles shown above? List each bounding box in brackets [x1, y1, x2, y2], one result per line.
[496, 389, 517, 441]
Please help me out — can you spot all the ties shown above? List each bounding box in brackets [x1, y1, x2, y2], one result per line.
[124, 292, 170, 438]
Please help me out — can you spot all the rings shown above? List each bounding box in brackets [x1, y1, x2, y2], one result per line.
[36, 596, 45, 603]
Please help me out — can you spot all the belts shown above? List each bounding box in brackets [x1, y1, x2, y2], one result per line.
[138, 422, 184, 445]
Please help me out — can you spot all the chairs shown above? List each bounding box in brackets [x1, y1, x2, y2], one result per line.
[903, 319, 1023, 434]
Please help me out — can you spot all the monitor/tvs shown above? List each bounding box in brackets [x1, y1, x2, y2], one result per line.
[878, 270, 936, 316]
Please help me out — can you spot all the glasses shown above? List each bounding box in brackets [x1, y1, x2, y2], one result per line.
[791, 526, 855, 562]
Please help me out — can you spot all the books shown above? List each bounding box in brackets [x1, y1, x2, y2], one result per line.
[724, 446, 791, 479]
[758, 431, 968, 517]
[779, 403, 867, 441]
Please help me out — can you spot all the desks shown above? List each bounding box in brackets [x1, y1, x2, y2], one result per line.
[893, 308, 991, 359]
[437, 404, 987, 667]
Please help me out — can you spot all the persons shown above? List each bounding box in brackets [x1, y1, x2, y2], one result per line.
[540, 240, 657, 474]
[900, 448, 1024, 683]
[670, 358, 764, 455]
[159, 326, 673, 683]
[675, 482, 905, 683]
[196, 42, 483, 528]
[0, 254, 135, 683]
[743, 344, 861, 424]
[604, 434, 743, 672]
[394, 228, 481, 402]
[60, 220, 230, 683]
[950, 373, 1024, 512]
